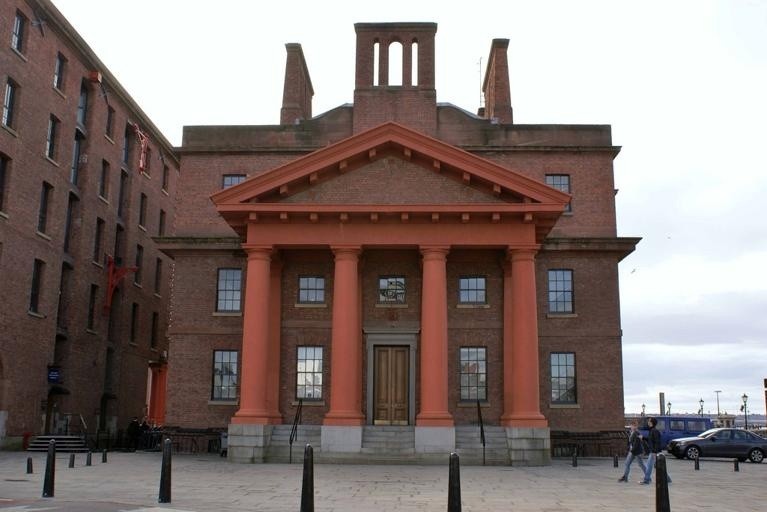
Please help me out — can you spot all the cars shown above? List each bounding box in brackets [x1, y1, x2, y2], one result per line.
[667, 427, 767, 463]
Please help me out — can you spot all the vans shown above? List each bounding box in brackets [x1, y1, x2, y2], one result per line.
[627, 415, 714, 454]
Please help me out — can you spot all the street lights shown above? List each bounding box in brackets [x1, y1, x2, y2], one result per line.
[741, 392, 749, 431]
[714, 390, 723, 414]
[642, 403, 646, 416]
[699, 398, 705, 416]
[667, 402, 672, 416]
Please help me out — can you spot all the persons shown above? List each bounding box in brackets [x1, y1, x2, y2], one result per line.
[638, 417, 673, 485]
[617, 421, 653, 484]
[126, 417, 142, 452]
